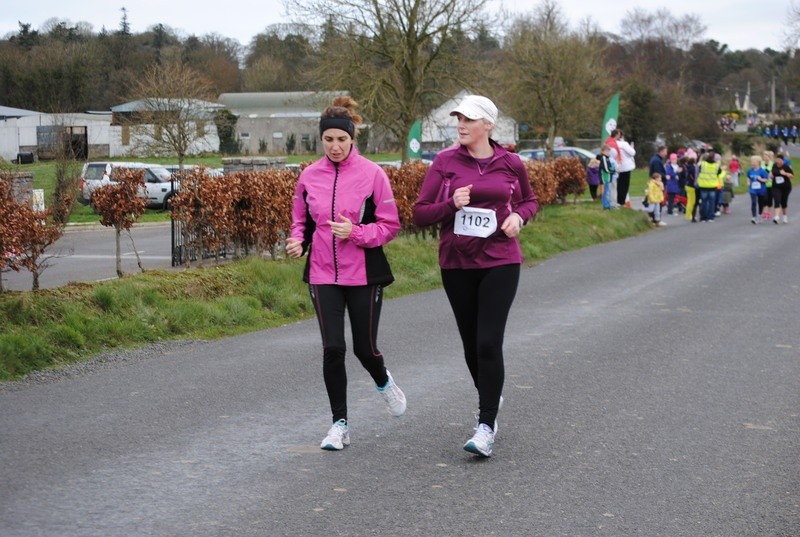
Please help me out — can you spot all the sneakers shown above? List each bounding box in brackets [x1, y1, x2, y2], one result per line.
[374, 369, 406, 417]
[462, 423, 494, 457]
[320, 419, 351, 449]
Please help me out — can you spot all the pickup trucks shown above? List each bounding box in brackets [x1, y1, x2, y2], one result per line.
[75, 161, 186, 211]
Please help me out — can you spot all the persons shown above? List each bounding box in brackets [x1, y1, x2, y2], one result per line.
[282, 97, 407, 452]
[408, 92, 540, 458]
[586, 123, 799, 225]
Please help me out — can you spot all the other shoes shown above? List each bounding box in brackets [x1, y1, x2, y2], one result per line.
[584, 193, 789, 226]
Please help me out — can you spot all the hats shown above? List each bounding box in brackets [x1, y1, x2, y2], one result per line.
[450, 95, 499, 125]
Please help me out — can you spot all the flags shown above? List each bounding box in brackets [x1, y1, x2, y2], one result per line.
[599, 91, 621, 140]
[406, 119, 421, 157]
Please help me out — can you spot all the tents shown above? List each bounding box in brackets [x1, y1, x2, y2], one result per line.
[410, 89, 520, 147]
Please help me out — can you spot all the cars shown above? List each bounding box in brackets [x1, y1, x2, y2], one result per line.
[520, 148, 600, 162]
[162, 162, 224, 180]
